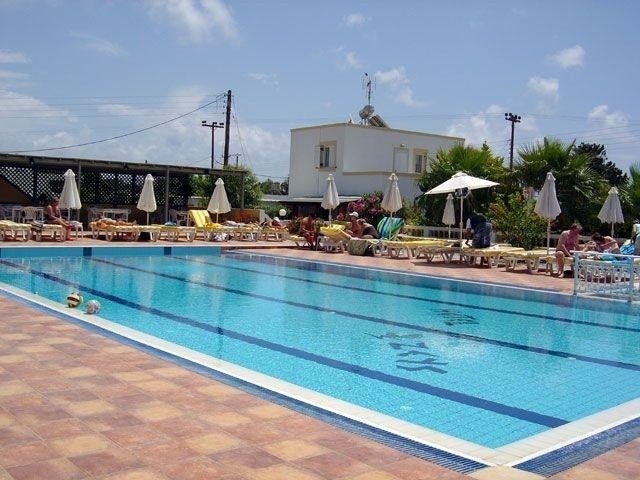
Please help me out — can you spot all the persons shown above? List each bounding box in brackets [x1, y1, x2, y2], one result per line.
[99, 215, 136, 226]
[356, 218, 378, 239]
[300, 212, 321, 250]
[347, 211, 359, 237]
[555, 222, 584, 278]
[465, 212, 490, 249]
[47, 197, 74, 241]
[258, 216, 283, 229]
[630, 218, 640, 256]
[591, 232, 623, 260]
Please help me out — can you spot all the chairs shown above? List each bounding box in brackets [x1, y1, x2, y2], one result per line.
[0, 217, 33, 242]
[91, 207, 163, 242]
[153, 222, 197, 242]
[22, 203, 85, 242]
[187, 209, 292, 241]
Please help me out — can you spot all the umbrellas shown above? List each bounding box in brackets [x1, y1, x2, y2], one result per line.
[533, 172, 562, 273]
[320, 174, 340, 227]
[441, 193, 456, 238]
[136, 174, 157, 226]
[207, 177, 231, 223]
[597, 187, 625, 239]
[380, 174, 403, 241]
[424, 172, 501, 248]
[58, 169, 82, 221]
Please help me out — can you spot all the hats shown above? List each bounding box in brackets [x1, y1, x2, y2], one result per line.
[349, 211, 359, 217]
[274, 217, 280, 223]
